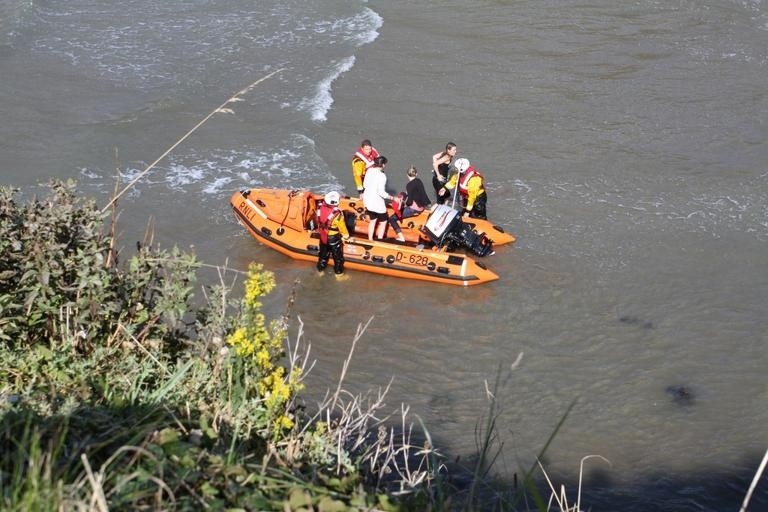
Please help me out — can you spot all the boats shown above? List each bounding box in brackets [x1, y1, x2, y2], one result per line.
[231, 188, 515, 285]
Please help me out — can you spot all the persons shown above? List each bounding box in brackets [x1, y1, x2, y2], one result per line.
[432, 142, 456, 205]
[389, 167, 430, 242]
[362, 156, 393, 241]
[438, 158, 487, 220]
[318, 190, 350, 281]
[352, 140, 380, 199]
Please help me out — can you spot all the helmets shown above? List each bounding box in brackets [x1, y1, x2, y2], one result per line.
[325, 191, 340, 205]
[455, 158, 470, 173]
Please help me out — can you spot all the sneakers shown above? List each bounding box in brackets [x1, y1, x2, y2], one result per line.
[319, 271, 324, 276]
[334, 273, 352, 281]
